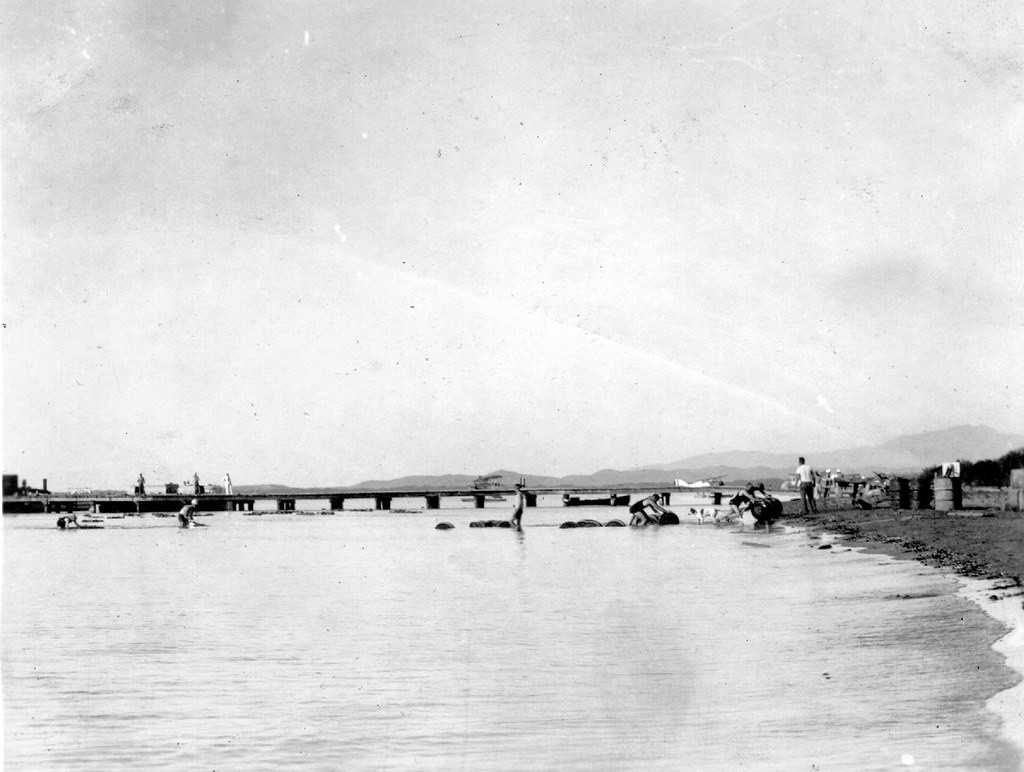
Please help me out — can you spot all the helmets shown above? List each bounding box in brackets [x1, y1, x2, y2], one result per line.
[191, 499, 198, 504]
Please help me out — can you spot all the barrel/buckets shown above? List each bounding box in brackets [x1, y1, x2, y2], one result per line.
[560, 519, 626, 529]
[889, 479, 927, 510]
[751, 497, 783, 520]
[470, 520, 510, 527]
[435, 521, 454, 529]
[659, 512, 679, 525]
[934, 477, 963, 511]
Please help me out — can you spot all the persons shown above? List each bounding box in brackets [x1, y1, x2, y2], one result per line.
[510, 484, 526, 532]
[711, 481, 724, 520]
[178, 499, 200, 529]
[57, 515, 81, 530]
[193, 472, 200, 496]
[629, 493, 674, 526]
[223, 473, 233, 495]
[136, 473, 145, 494]
[796, 457, 851, 514]
[719, 482, 776, 524]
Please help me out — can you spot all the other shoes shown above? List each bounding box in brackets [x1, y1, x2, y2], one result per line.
[804, 511, 809, 515]
[812, 510, 818, 514]
